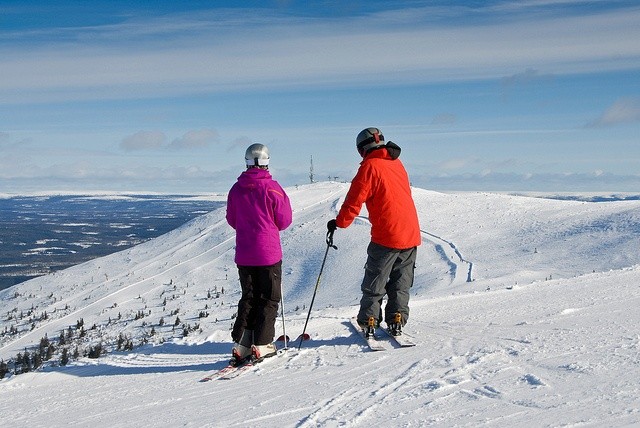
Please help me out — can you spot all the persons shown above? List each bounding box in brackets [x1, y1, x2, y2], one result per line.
[327, 128, 421, 327]
[226, 144, 293, 359]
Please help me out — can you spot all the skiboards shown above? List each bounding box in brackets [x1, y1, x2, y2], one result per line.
[198, 333, 310, 382]
[350, 316, 416, 351]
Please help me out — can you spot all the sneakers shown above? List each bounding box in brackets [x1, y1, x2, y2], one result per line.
[357, 318, 377, 333]
[251, 344, 278, 359]
[387, 313, 402, 336]
[232, 343, 252, 360]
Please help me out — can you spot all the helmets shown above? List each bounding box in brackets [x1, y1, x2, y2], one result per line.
[246, 144, 270, 166]
[356, 128, 385, 157]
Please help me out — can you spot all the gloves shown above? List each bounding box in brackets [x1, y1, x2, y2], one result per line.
[327, 220, 337, 232]
